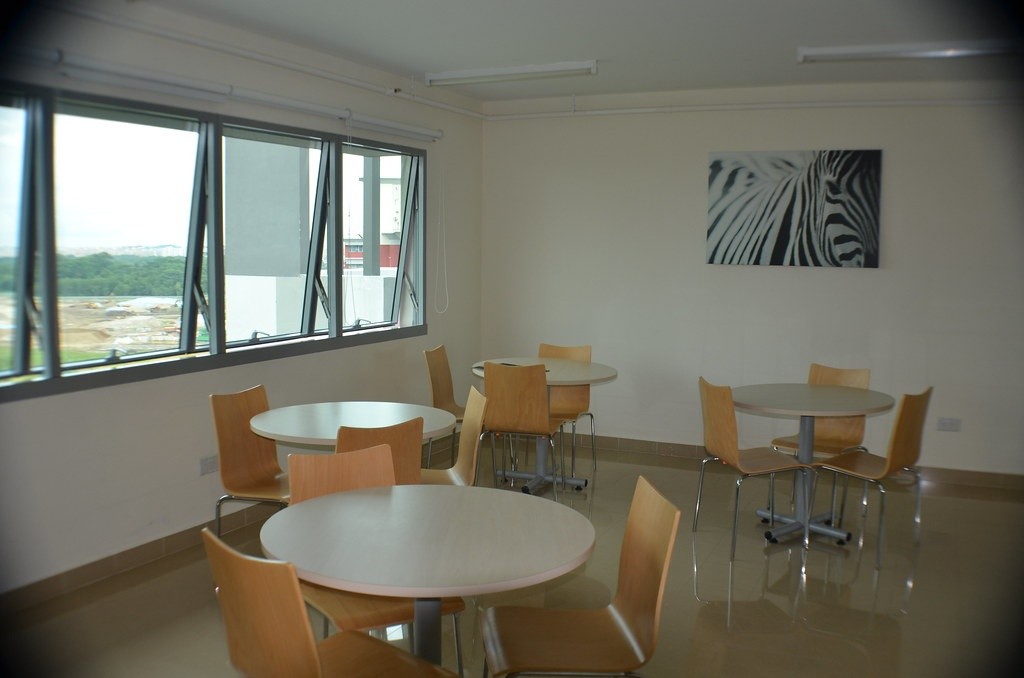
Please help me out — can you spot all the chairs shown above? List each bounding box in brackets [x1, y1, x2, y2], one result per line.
[336, 417, 424, 486]
[809, 385, 933, 569]
[288, 443, 466, 676]
[483, 361, 566, 502]
[766, 363, 871, 532]
[209, 384, 290, 539]
[481, 473, 682, 678]
[422, 344, 516, 488]
[420, 384, 488, 486]
[691, 375, 819, 563]
[525, 343, 597, 478]
[198, 527, 461, 677]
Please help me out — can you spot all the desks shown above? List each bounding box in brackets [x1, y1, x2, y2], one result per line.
[250, 401, 456, 452]
[733, 381, 895, 545]
[472, 356, 617, 495]
[260, 484, 598, 665]
